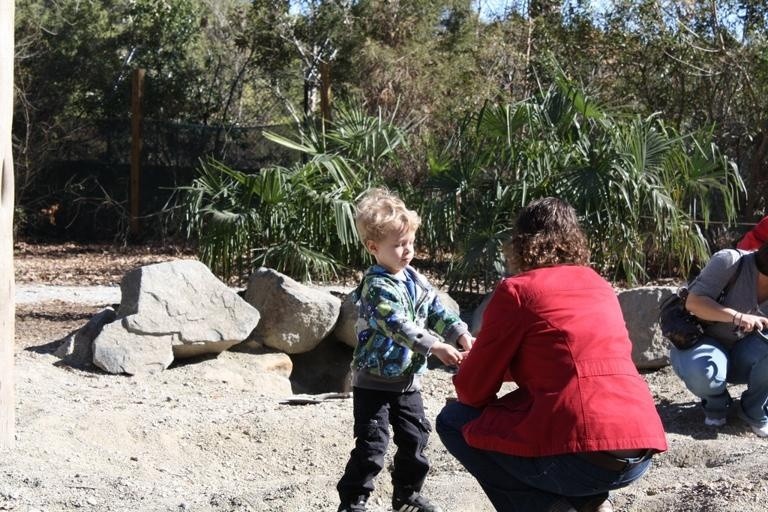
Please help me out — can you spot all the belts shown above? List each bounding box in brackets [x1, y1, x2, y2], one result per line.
[581, 451, 653, 473]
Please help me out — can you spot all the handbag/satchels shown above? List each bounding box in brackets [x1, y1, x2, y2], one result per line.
[658, 294, 709, 351]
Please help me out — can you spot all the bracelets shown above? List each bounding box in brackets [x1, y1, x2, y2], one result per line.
[733, 312, 743, 328]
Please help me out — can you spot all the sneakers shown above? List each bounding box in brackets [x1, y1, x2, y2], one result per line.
[561, 498, 613, 512]
[748, 424, 768, 437]
[704, 416, 727, 428]
[336, 501, 367, 512]
[391, 492, 434, 512]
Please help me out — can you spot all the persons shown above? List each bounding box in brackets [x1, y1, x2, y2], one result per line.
[436, 197, 668, 512]
[670, 245, 768, 438]
[337, 197, 477, 512]
